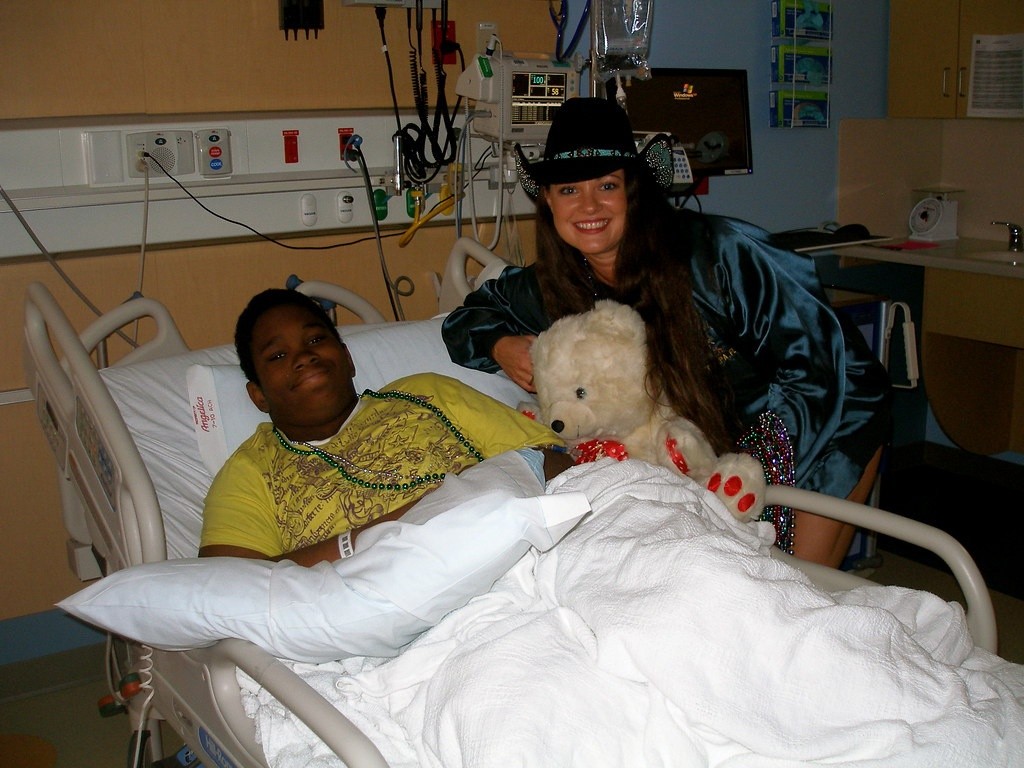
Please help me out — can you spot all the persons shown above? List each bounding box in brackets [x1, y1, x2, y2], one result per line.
[198, 288, 578, 568]
[440, 97, 894, 570]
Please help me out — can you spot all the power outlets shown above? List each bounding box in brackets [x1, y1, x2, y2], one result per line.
[126, 130, 196, 179]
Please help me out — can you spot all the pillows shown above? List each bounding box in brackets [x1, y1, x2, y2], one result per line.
[184, 312, 540, 476]
[49, 447, 592, 667]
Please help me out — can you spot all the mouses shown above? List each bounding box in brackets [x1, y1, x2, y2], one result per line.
[833, 224, 870, 240]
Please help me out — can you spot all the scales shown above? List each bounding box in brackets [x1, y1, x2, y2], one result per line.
[908, 185, 965, 242]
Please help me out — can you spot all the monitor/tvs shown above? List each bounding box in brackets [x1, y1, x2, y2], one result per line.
[604, 68, 753, 176]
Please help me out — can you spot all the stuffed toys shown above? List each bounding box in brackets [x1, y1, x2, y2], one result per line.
[516, 299, 766, 523]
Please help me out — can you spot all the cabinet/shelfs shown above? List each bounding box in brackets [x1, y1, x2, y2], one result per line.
[887, 1, 1024, 119]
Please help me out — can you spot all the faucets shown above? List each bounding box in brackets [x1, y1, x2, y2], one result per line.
[990, 221, 1023, 252]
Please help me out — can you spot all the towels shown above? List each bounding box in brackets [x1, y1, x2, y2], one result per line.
[880, 240, 940, 251]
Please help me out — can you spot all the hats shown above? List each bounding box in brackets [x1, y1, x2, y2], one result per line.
[512, 97, 674, 205]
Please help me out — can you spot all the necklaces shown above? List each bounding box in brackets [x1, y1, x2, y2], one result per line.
[582, 255, 601, 304]
[273, 388, 485, 490]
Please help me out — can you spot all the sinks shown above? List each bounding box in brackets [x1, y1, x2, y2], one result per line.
[962, 250, 1024, 264]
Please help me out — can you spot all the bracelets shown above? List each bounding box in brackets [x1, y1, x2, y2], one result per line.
[337, 530, 353, 559]
[731, 411, 796, 555]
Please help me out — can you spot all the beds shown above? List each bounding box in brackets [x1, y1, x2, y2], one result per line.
[21, 237, 1024, 768]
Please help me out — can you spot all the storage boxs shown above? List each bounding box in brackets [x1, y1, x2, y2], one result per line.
[769, 89, 831, 129]
[770, 44, 833, 85]
[770, 0, 834, 40]
